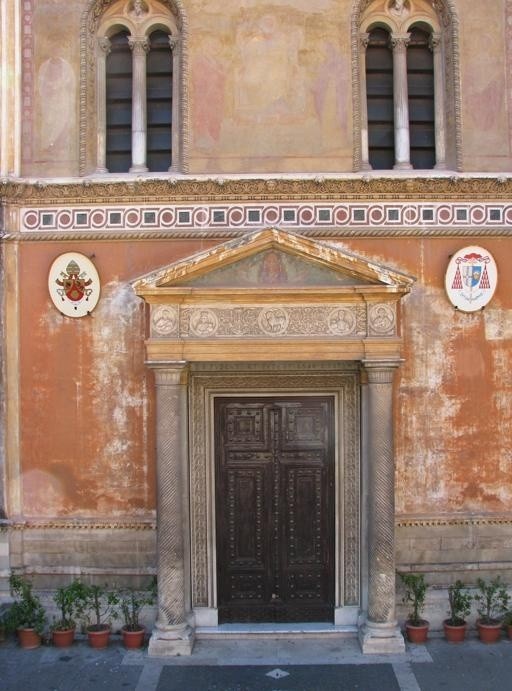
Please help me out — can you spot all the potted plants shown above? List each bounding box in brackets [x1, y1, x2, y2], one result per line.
[395, 568, 512, 645]
[0, 570, 157, 651]
